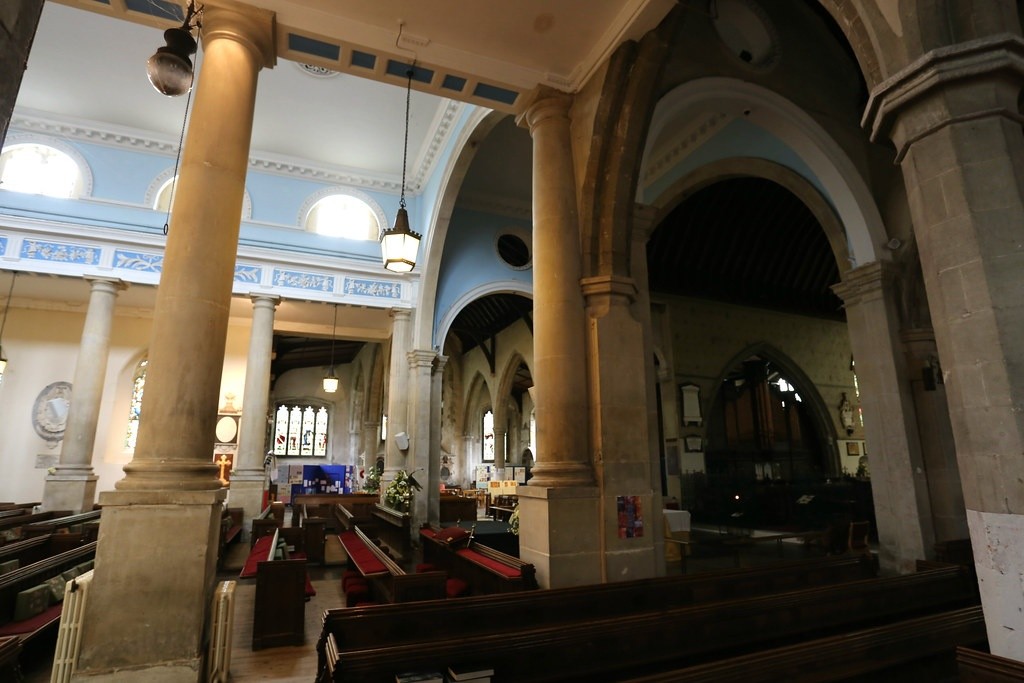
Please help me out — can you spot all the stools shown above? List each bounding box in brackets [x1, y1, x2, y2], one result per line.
[342, 531, 469, 608]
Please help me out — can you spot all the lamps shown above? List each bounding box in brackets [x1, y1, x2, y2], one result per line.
[323, 303, 339, 393]
[0, 269, 17, 382]
[147, 0, 198, 98]
[380, 70, 422, 273]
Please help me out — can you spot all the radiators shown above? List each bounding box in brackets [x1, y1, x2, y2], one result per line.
[206, 579, 237, 683]
[49, 569, 95, 683]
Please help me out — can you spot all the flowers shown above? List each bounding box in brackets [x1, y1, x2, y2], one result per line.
[506, 510, 520, 535]
[363, 466, 424, 509]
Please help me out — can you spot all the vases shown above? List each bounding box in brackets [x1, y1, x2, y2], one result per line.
[384, 500, 409, 513]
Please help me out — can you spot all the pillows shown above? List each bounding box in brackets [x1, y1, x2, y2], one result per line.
[433, 526, 471, 544]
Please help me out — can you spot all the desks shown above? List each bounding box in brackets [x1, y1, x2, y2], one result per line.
[420, 527, 536, 594]
[315, 551, 987, 682]
[662, 508, 691, 562]
[0, 502, 406, 683]
[293, 494, 380, 507]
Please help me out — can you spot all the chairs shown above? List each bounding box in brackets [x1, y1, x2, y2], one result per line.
[848, 520, 869, 550]
